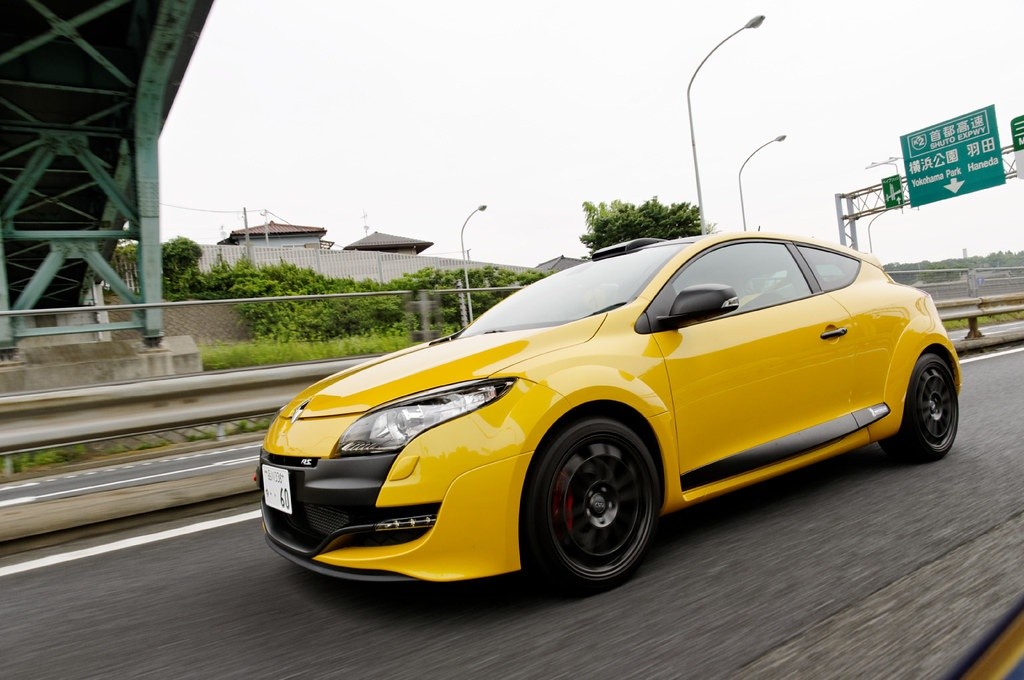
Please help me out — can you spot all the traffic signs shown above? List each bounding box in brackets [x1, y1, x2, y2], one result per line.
[897, 104, 1006, 208]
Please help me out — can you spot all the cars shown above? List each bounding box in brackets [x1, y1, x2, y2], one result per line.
[253, 234, 966, 584]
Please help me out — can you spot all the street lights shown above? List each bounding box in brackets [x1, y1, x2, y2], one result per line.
[687, 14, 767, 233]
[738, 134, 786, 230]
[461, 204, 488, 323]
